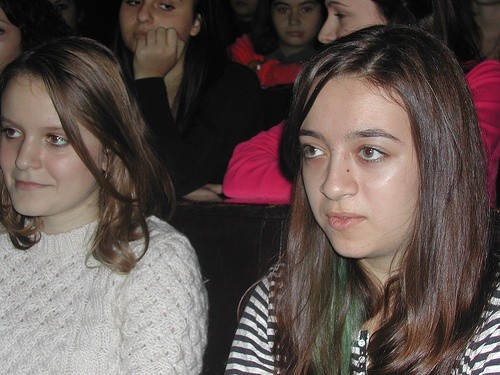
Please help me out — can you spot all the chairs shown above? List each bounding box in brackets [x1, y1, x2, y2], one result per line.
[160, 204, 289, 375]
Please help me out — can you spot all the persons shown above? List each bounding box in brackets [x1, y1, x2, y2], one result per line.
[223, 0, 500, 207]
[225, 0, 328, 86]
[224, 25, 499, 375]
[0, 0, 84, 68]
[97, 0, 271, 201]
[0, 35, 210, 374]
[229, 0, 261, 23]
